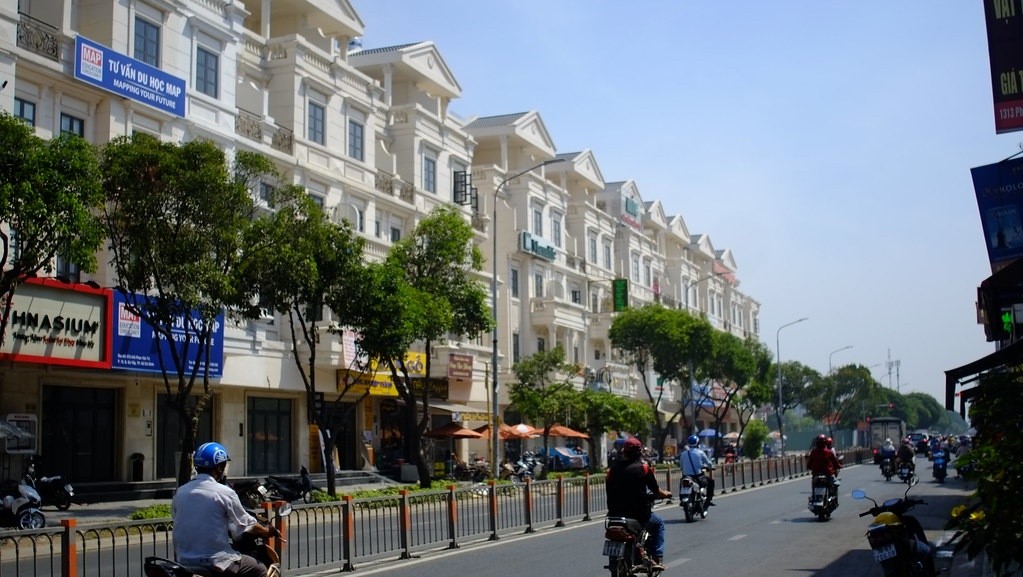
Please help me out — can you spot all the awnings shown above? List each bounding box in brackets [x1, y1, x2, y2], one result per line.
[399, 398, 493, 421]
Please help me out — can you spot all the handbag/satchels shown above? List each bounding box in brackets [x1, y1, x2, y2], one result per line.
[696, 471, 708, 488]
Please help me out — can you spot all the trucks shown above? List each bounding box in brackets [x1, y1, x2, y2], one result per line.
[869, 417, 906, 464]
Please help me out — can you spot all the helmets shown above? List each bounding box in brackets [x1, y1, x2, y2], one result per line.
[901, 438, 909, 444]
[816, 434, 826, 444]
[686, 434, 700, 447]
[193, 442, 231, 469]
[826, 437, 833, 444]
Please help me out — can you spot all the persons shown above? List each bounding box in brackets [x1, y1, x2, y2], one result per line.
[170, 443, 268, 577]
[879, 438, 896, 468]
[679, 434, 717, 507]
[920, 436, 973, 479]
[605, 446, 673, 569]
[806, 435, 845, 491]
[897, 437, 915, 472]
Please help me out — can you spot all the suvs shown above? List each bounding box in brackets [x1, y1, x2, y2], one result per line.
[906, 432, 928, 453]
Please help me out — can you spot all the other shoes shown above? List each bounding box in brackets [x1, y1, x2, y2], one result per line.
[704, 500, 716, 506]
[651, 561, 667, 570]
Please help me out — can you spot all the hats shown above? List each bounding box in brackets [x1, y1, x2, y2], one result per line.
[622, 438, 642, 455]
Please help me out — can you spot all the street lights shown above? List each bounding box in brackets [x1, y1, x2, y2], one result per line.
[829, 343, 856, 407]
[683, 269, 737, 434]
[775, 316, 809, 457]
[491, 156, 574, 476]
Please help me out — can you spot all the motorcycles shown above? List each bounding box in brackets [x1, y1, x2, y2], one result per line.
[928, 448, 948, 483]
[805, 453, 848, 521]
[142, 486, 289, 577]
[897, 461, 916, 484]
[679, 466, 716, 523]
[263, 465, 313, 503]
[19, 455, 79, 512]
[602, 488, 676, 577]
[218, 475, 271, 508]
[880, 459, 895, 481]
[852, 473, 940, 577]
[0, 475, 46, 530]
[471, 446, 550, 485]
[955, 455, 973, 482]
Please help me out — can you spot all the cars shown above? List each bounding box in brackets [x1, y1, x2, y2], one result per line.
[541, 445, 589, 471]
[641, 447, 660, 467]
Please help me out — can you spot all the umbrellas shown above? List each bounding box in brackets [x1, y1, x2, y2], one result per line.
[723, 431, 743, 438]
[697, 429, 722, 438]
[767, 431, 788, 439]
[453, 423, 534, 473]
[422, 422, 483, 473]
[527, 425, 590, 470]
[511, 425, 540, 462]
[608, 430, 642, 450]
